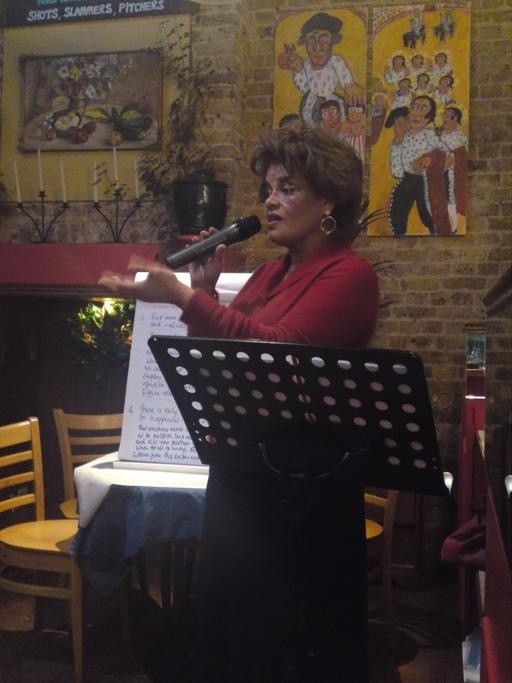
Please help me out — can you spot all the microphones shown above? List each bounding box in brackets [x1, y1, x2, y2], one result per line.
[165, 215, 262, 270]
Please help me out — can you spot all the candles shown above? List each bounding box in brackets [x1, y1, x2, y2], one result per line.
[59, 158, 68, 203]
[93, 160, 99, 203]
[134, 159, 139, 199]
[113, 146, 119, 191]
[13, 161, 22, 203]
[37, 149, 45, 191]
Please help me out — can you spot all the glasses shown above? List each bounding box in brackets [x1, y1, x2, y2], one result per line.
[257, 182, 297, 205]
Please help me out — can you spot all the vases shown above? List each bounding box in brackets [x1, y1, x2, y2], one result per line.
[175, 180, 226, 236]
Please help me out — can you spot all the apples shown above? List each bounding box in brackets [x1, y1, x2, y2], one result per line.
[109, 131, 123, 147]
[72, 128, 88, 144]
[83, 121, 96, 134]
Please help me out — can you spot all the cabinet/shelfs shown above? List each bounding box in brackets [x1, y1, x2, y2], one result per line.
[0, 244, 235, 300]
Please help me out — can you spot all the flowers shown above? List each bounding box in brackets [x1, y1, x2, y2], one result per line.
[93, 13, 222, 260]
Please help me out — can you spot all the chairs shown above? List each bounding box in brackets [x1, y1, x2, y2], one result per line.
[51, 408, 123, 519]
[0, 417, 132, 683]
[364, 490, 398, 644]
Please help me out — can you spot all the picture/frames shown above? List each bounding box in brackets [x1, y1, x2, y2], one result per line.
[19, 46, 163, 151]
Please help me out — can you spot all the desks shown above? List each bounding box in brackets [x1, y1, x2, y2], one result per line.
[69, 450, 210, 626]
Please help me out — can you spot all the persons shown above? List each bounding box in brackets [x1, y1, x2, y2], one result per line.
[383, 107, 407, 180]
[431, 50, 452, 78]
[411, 53, 427, 72]
[312, 99, 341, 138]
[391, 96, 449, 232]
[436, 108, 466, 219]
[98, 124, 377, 681]
[395, 78, 411, 94]
[280, 113, 304, 139]
[383, 54, 406, 81]
[414, 72, 429, 91]
[340, 86, 367, 154]
[435, 71, 455, 98]
[280, 14, 365, 139]
